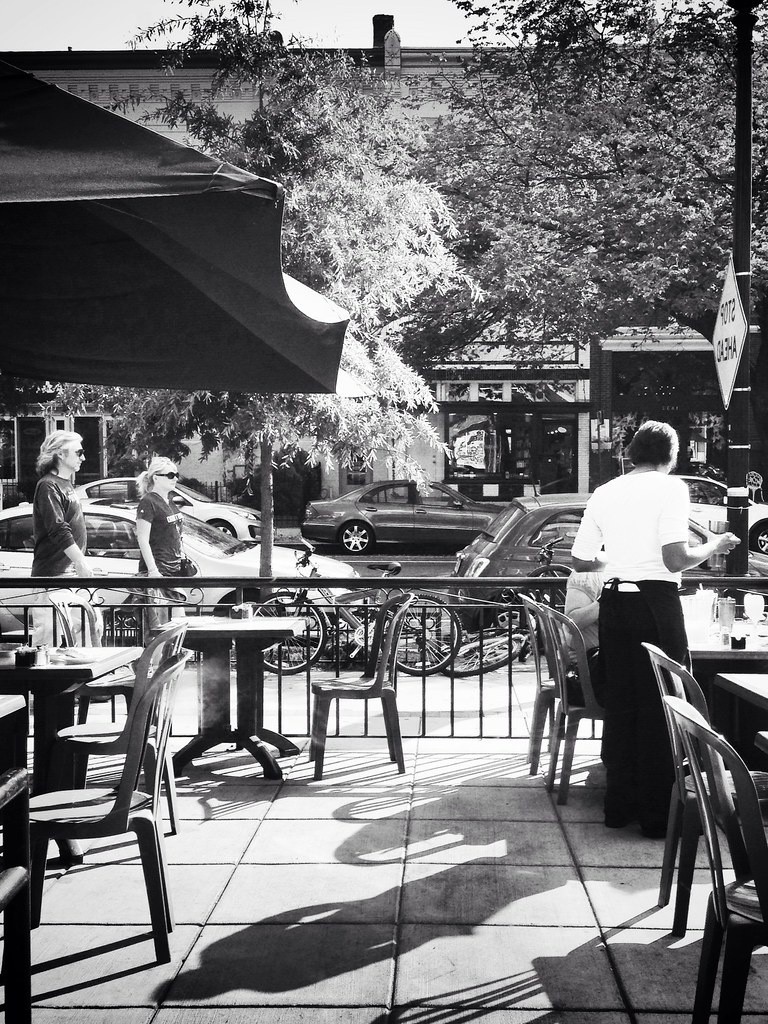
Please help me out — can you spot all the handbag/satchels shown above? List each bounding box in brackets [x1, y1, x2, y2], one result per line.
[181, 559, 198, 577]
[564, 661, 603, 707]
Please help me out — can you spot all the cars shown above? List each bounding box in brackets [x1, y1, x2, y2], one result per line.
[0, 477, 361, 639]
[301, 479, 506, 553]
[450, 475, 768, 631]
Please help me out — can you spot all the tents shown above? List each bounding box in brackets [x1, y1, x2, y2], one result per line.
[0, 56, 354, 394]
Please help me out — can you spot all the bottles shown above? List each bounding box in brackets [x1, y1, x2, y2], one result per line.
[242, 604, 253, 619]
[36, 644, 50, 666]
[721, 625, 729, 650]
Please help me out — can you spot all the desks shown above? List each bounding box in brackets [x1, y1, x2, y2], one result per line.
[0, 642, 146, 868]
[677, 594, 768, 751]
[149, 616, 315, 780]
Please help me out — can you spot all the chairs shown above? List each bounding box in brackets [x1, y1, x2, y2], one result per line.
[746, 471, 764, 502]
[85, 521, 118, 549]
[29, 650, 191, 962]
[311, 592, 416, 780]
[659, 694, 768, 1024]
[53, 592, 190, 837]
[640, 641, 768, 937]
[519, 592, 604, 805]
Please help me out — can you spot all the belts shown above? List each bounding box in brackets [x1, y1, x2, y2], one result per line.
[603, 582, 640, 592]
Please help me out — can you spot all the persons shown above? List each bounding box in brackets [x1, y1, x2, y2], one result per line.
[562, 572, 604, 767]
[571, 419, 741, 842]
[29, 428, 113, 710]
[136, 456, 185, 666]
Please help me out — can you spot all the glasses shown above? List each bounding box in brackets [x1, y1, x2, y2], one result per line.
[156, 472, 179, 479]
[64, 449, 85, 457]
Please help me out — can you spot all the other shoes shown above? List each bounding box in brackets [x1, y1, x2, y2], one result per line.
[604, 792, 638, 829]
[636, 806, 705, 838]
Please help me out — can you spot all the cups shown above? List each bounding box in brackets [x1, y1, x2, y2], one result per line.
[694, 590, 735, 633]
[708, 520, 729, 568]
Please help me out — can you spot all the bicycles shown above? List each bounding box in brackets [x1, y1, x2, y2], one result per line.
[253, 538, 572, 678]
